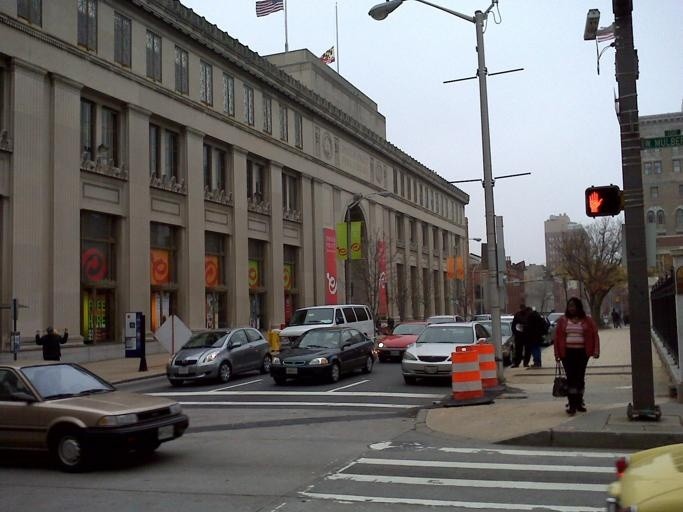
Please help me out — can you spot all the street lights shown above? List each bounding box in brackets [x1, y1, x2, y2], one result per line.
[368, 0, 509, 391]
[454, 237, 482, 314]
[346, 191, 394, 304]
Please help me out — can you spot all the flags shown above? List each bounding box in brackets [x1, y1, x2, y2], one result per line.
[256, 1, 284, 18]
[320, 46, 336, 64]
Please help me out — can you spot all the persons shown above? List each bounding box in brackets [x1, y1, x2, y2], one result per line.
[510, 304, 531, 368]
[526, 306, 541, 367]
[36, 326, 69, 361]
[601, 307, 622, 329]
[554, 298, 600, 414]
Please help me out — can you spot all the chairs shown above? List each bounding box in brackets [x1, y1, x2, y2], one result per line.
[0, 371, 28, 401]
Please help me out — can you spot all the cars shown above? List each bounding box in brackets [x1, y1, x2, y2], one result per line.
[0, 361, 190, 473]
[605, 442, 683, 512]
[270, 327, 377, 385]
[378, 311, 568, 385]
[167, 326, 272, 387]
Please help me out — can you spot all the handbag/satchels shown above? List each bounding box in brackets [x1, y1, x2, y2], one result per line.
[552, 360, 567, 397]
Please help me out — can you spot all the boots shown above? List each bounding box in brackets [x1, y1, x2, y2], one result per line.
[566, 393, 577, 414]
[576, 393, 586, 412]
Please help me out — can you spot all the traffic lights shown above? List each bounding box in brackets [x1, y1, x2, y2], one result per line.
[585, 185, 622, 218]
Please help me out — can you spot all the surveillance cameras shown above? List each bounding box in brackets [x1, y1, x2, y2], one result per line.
[584, 9, 600, 40]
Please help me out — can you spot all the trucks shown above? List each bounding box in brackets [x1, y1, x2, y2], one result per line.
[280, 304, 375, 350]
